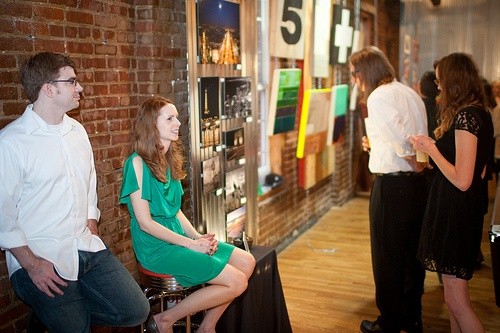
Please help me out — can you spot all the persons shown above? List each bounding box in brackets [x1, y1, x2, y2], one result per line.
[410, 52, 494, 333]
[417, 70, 441, 182]
[118, 96, 256, 333]
[0, 51, 150, 333]
[480, 76, 500, 261]
[349, 46, 433, 333]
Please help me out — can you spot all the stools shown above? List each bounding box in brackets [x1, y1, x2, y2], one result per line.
[133, 263, 207, 333]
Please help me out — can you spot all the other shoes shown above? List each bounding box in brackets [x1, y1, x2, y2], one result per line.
[143, 317, 160, 333]
[360, 319, 424, 333]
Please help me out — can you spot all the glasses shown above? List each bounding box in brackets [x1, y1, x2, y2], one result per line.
[351, 70, 360, 78]
[47, 77, 77, 86]
[434, 79, 441, 86]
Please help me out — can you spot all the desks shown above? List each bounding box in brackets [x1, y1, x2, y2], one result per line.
[164, 244, 293, 333]
